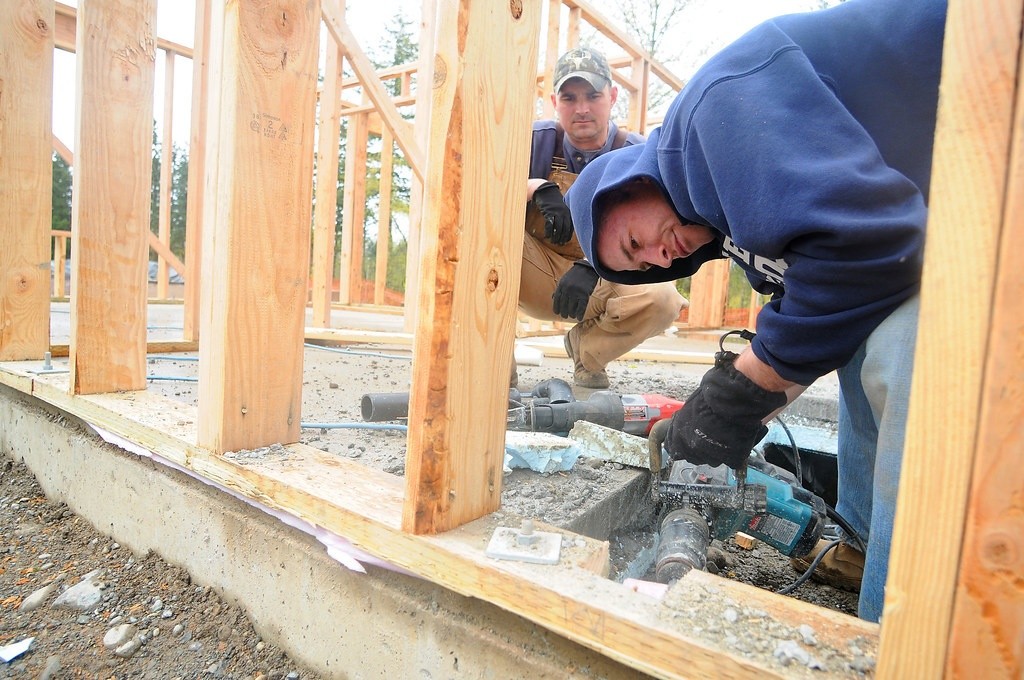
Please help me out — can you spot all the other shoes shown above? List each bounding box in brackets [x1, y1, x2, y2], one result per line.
[564, 331, 609, 388]
[510, 372, 519, 387]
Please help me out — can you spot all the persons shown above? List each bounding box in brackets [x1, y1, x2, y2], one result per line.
[567, 0, 949, 627]
[509, 46, 689, 392]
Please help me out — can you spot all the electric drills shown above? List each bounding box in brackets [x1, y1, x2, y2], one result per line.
[528, 392, 686, 433]
[648, 418, 827, 585]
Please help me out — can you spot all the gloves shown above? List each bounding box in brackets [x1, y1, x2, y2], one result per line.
[665, 359, 788, 469]
[532, 182, 574, 245]
[551, 260, 599, 321]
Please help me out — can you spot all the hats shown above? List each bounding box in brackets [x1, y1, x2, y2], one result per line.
[552, 47, 611, 96]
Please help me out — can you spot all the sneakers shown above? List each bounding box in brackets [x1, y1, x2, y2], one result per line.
[791, 538, 865, 592]
